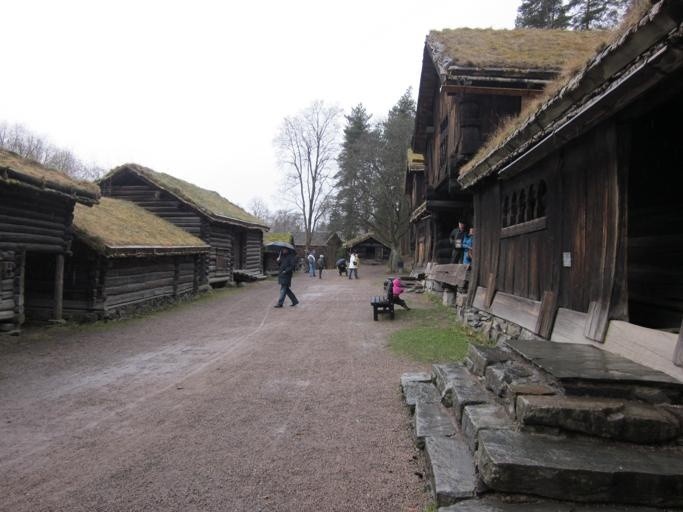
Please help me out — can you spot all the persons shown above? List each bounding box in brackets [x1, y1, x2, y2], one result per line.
[337, 262, 349, 276]
[348, 250, 360, 280]
[354, 252, 361, 272]
[387, 277, 411, 311]
[461, 225, 474, 266]
[316, 254, 327, 280]
[449, 218, 468, 264]
[307, 252, 317, 277]
[274, 246, 299, 308]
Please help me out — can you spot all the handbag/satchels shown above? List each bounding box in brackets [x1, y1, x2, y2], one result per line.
[354, 261, 357, 265]
[454, 239, 460, 248]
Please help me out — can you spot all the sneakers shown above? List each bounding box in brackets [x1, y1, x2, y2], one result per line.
[275, 304, 281, 307]
[290, 300, 298, 306]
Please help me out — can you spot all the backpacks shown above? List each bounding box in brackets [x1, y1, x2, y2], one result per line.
[308, 255, 314, 264]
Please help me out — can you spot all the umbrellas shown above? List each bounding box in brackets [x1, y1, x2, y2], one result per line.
[265, 240, 299, 258]
[335, 258, 345, 265]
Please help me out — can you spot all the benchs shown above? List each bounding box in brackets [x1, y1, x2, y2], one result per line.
[372, 279, 394, 321]
